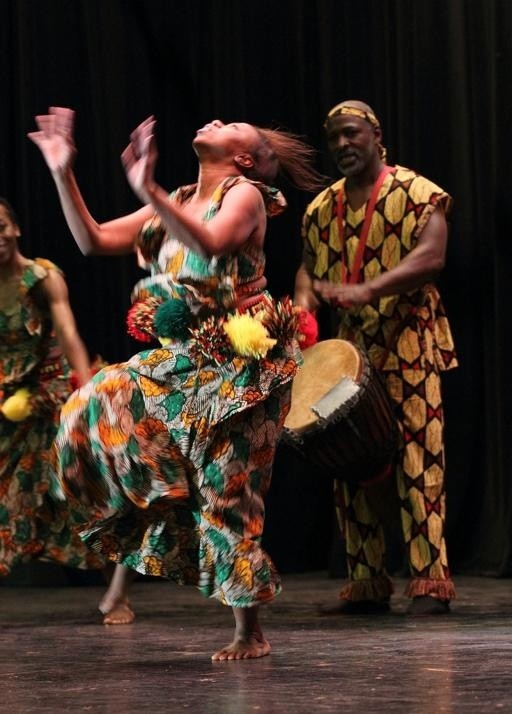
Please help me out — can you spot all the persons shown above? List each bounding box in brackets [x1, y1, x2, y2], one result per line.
[1, 202, 136, 627]
[27, 103, 323, 662]
[291, 98, 460, 621]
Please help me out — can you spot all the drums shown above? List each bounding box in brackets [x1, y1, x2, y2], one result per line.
[284, 338, 405, 488]
